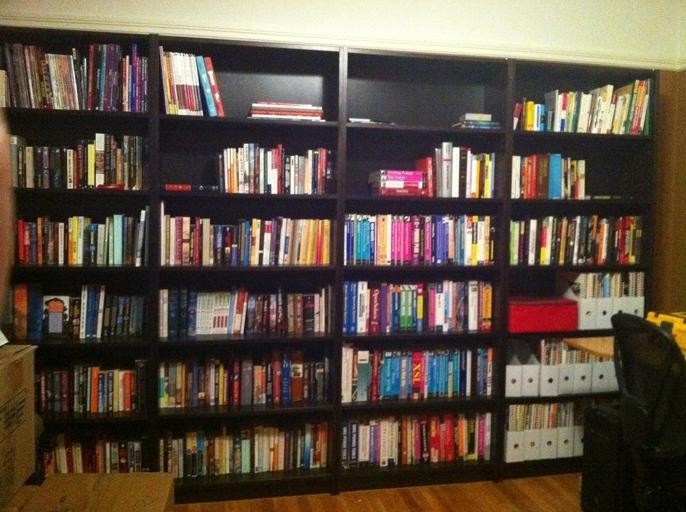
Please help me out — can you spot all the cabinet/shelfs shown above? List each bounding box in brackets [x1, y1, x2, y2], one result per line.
[500, 59, 660, 481]
[0, 24, 154, 477]
[154, 33, 343, 504]
[343, 47, 512, 492]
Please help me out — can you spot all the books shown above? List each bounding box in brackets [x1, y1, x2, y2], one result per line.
[159, 355, 335, 482]
[245, 99, 327, 123]
[217, 141, 337, 198]
[513, 77, 650, 137]
[451, 112, 501, 131]
[158, 198, 335, 339]
[368, 141, 499, 199]
[511, 153, 585, 200]
[339, 345, 498, 472]
[559, 272, 645, 330]
[508, 215, 645, 264]
[339, 212, 498, 335]
[33, 361, 150, 476]
[503, 337, 618, 395]
[504, 403, 584, 463]
[346, 118, 396, 126]
[1, 39, 151, 189]
[14, 204, 150, 344]
[158, 44, 226, 118]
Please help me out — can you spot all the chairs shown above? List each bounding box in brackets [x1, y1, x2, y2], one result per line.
[613, 315, 686, 512]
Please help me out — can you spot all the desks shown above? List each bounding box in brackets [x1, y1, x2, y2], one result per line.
[0, 473, 175, 512]
[564, 336, 613, 359]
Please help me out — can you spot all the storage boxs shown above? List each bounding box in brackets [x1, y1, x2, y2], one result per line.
[506, 296, 578, 333]
[0, 345, 36, 504]
